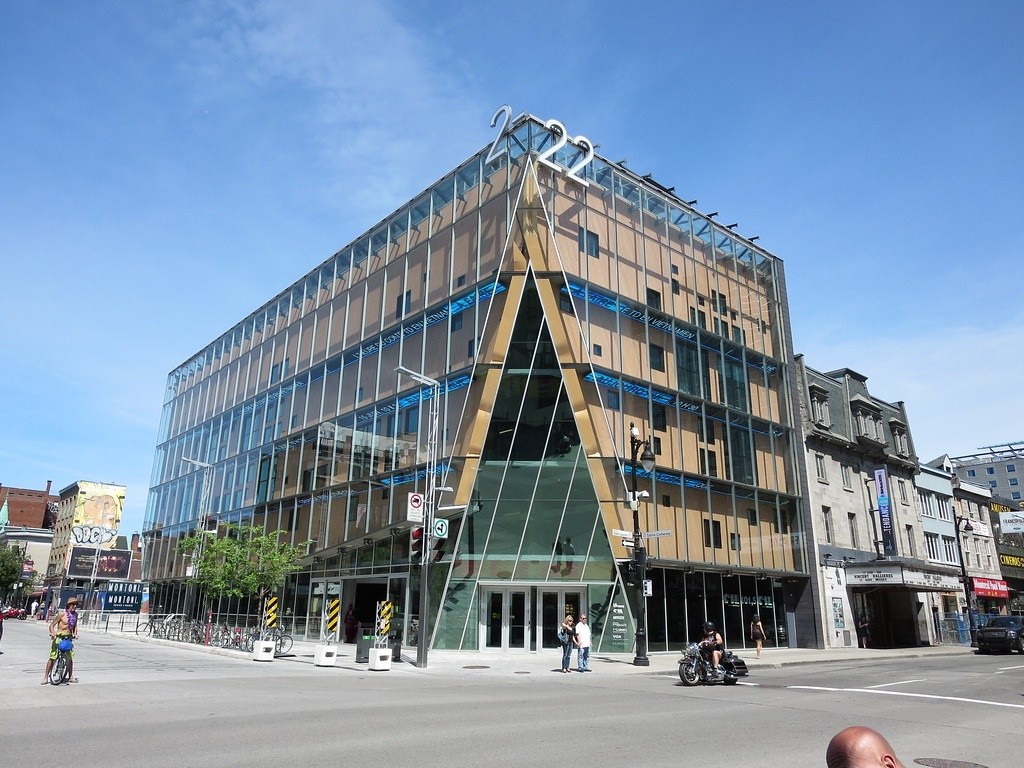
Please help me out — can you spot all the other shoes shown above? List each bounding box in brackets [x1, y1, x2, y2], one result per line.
[713, 667, 718, 673]
[43, 676, 48, 684]
[578, 667, 583, 672]
[584, 667, 592, 671]
[567, 668, 571, 672]
[562, 669, 567, 673]
[69, 677, 78, 684]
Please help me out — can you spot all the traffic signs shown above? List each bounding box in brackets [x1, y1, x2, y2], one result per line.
[621, 539, 634, 548]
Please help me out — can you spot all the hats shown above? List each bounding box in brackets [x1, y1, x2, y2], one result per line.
[66, 598, 79, 605]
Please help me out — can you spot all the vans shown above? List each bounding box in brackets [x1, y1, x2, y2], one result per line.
[390, 612, 420, 637]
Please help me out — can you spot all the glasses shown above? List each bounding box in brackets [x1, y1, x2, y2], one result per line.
[583, 618, 587, 620]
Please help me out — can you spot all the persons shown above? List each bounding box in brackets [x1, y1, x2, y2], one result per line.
[561, 615, 576, 673]
[751, 614, 766, 657]
[344, 605, 355, 641]
[859, 616, 869, 648]
[41, 597, 78, 684]
[573, 614, 593, 672]
[700, 621, 724, 675]
[826, 726, 906, 768]
[31, 599, 39, 618]
[40, 601, 45, 614]
[553, 536, 575, 569]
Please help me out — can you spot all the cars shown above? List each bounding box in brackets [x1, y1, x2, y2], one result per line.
[976, 615, 1024, 654]
[3, 608, 28, 620]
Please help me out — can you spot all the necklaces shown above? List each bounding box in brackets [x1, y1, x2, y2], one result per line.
[66, 608, 77, 628]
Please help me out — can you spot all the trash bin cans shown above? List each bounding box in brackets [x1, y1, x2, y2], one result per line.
[38, 606, 45, 620]
[354, 620, 404, 663]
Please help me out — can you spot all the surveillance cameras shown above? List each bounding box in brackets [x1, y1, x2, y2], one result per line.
[642, 491, 650, 500]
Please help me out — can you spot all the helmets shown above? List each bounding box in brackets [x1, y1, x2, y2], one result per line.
[702, 621, 716, 629]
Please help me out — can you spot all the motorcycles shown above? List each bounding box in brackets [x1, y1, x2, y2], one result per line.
[678, 641, 747, 685]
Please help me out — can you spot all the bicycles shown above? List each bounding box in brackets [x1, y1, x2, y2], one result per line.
[50, 634, 78, 685]
[136, 614, 293, 654]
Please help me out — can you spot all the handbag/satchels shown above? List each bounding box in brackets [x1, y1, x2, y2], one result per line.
[558, 627, 569, 644]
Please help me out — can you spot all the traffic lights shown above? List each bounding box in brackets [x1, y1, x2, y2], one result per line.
[409, 525, 424, 563]
[429, 537, 445, 563]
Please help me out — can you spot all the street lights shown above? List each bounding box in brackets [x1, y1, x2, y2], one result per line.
[396, 364, 454, 669]
[629, 422, 656, 667]
[180, 455, 215, 617]
[952, 505, 980, 646]
